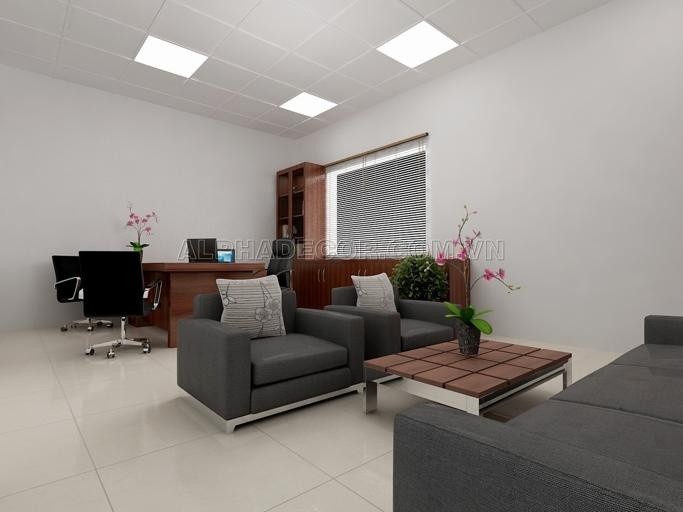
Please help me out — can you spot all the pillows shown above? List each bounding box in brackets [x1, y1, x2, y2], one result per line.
[216, 274, 287, 339]
[351, 273, 398, 313]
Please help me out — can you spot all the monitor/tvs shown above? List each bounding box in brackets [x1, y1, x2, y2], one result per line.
[186, 237, 217, 263]
[217, 249, 235, 263]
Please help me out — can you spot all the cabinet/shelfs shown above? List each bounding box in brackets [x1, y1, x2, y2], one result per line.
[276, 162, 326, 259]
[292, 259, 470, 311]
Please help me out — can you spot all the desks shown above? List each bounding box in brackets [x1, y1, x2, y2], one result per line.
[128, 263, 265, 348]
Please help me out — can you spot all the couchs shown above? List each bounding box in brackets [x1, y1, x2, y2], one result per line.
[176, 288, 364, 434]
[393, 315, 683, 512]
[323, 283, 461, 385]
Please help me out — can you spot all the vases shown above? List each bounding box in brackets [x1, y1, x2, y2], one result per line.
[456, 320, 481, 355]
[140, 252, 143, 262]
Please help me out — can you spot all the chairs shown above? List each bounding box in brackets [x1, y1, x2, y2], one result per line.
[78, 250, 151, 358]
[51, 255, 113, 332]
[250, 238, 296, 288]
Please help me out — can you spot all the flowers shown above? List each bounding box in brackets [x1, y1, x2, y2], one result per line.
[424, 205, 520, 335]
[126, 206, 159, 252]
